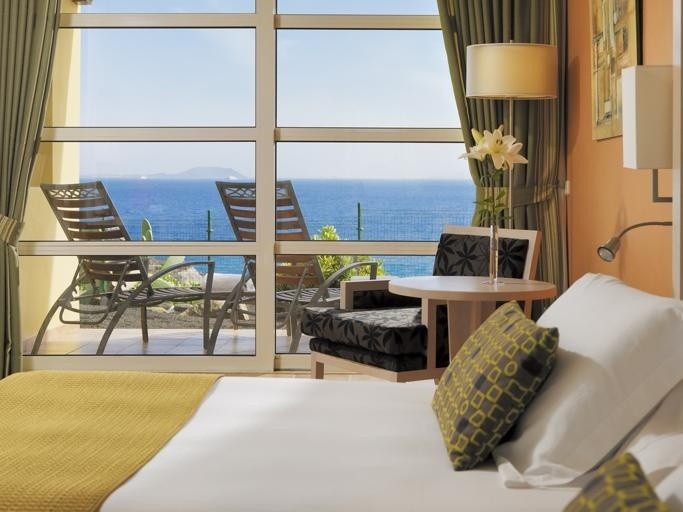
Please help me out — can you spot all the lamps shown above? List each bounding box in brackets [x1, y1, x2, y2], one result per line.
[596, 66, 672, 265]
[465, 42, 558, 231]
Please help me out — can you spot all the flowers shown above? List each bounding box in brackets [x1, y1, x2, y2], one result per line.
[457, 129, 529, 286]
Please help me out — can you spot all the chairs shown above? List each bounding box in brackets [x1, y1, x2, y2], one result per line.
[206, 174, 381, 357]
[29, 180, 255, 357]
[298, 224, 542, 384]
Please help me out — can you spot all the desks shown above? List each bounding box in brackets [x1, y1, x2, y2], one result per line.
[388, 275, 557, 365]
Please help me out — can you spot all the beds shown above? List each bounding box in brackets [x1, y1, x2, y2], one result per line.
[0, 368, 680, 510]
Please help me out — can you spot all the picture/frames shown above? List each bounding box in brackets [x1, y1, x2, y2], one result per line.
[587, 1, 643, 143]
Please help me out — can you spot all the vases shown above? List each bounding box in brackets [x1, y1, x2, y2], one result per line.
[488, 224, 498, 285]
